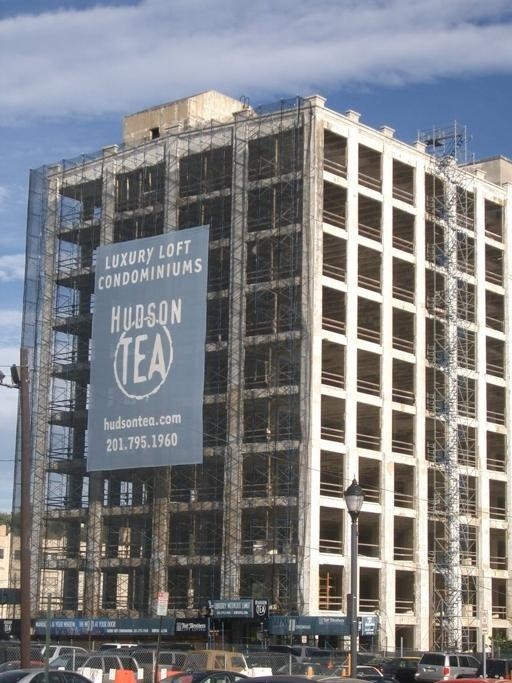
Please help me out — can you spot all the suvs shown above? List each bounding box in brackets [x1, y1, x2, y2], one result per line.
[414, 652, 482, 680]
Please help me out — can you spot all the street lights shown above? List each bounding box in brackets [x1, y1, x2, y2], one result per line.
[344, 473, 366, 678]
[0, 347, 35, 677]
[201, 600, 216, 647]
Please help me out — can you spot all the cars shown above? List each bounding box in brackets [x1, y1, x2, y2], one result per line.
[476, 658, 512, 680]
[1, 636, 373, 683]
[362, 657, 422, 681]
